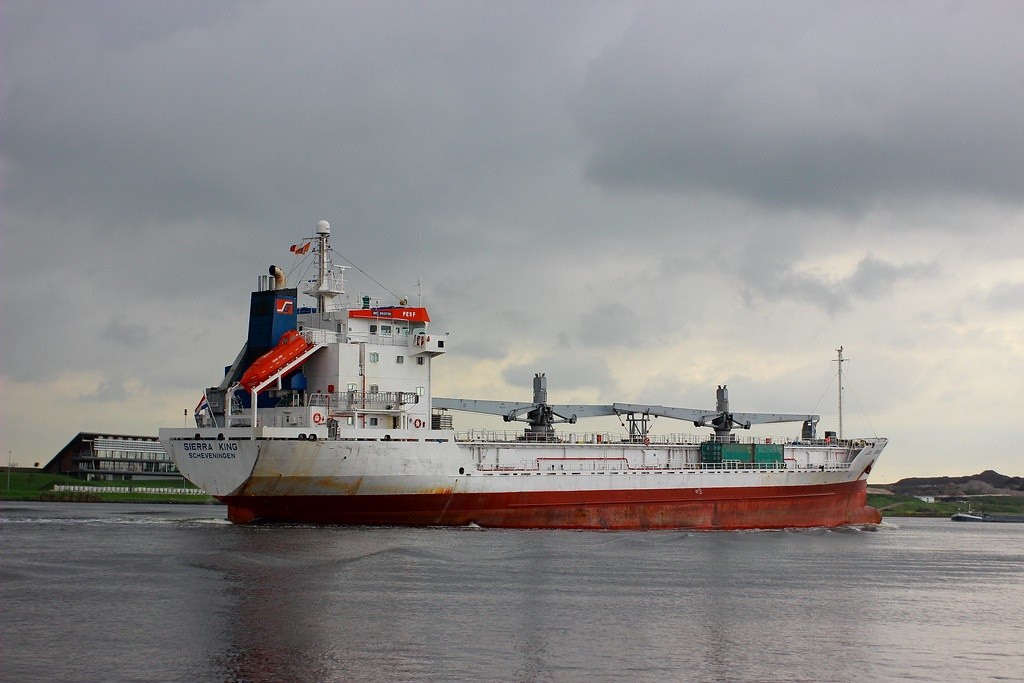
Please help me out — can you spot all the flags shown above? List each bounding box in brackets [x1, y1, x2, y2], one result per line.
[194, 394, 209, 417]
[290, 241, 311, 255]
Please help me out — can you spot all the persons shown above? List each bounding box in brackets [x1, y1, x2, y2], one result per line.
[817, 437, 830, 445]
[787, 438, 789, 446]
[795, 437, 799, 445]
[979, 511, 984, 517]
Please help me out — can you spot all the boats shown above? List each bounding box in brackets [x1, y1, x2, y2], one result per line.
[240, 329, 309, 394]
[159, 220, 889, 532]
[951, 499, 982, 522]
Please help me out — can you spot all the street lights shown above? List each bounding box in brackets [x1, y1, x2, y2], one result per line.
[7, 451, 11, 491]
